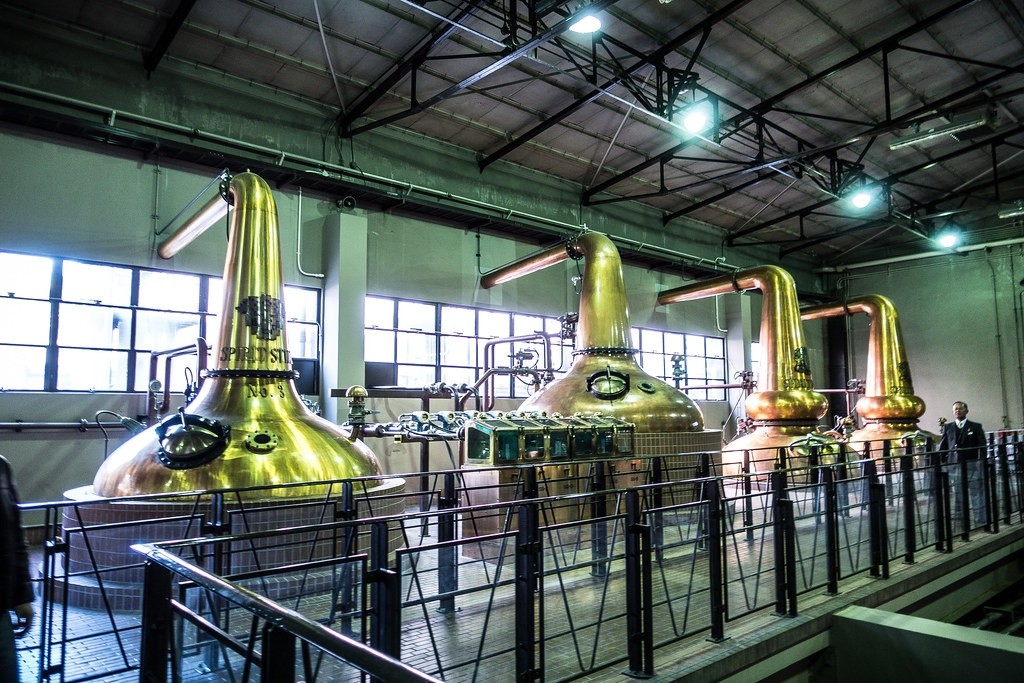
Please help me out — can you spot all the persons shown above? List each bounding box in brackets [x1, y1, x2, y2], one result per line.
[937, 401, 988, 529]
[1, 453, 36, 682]
